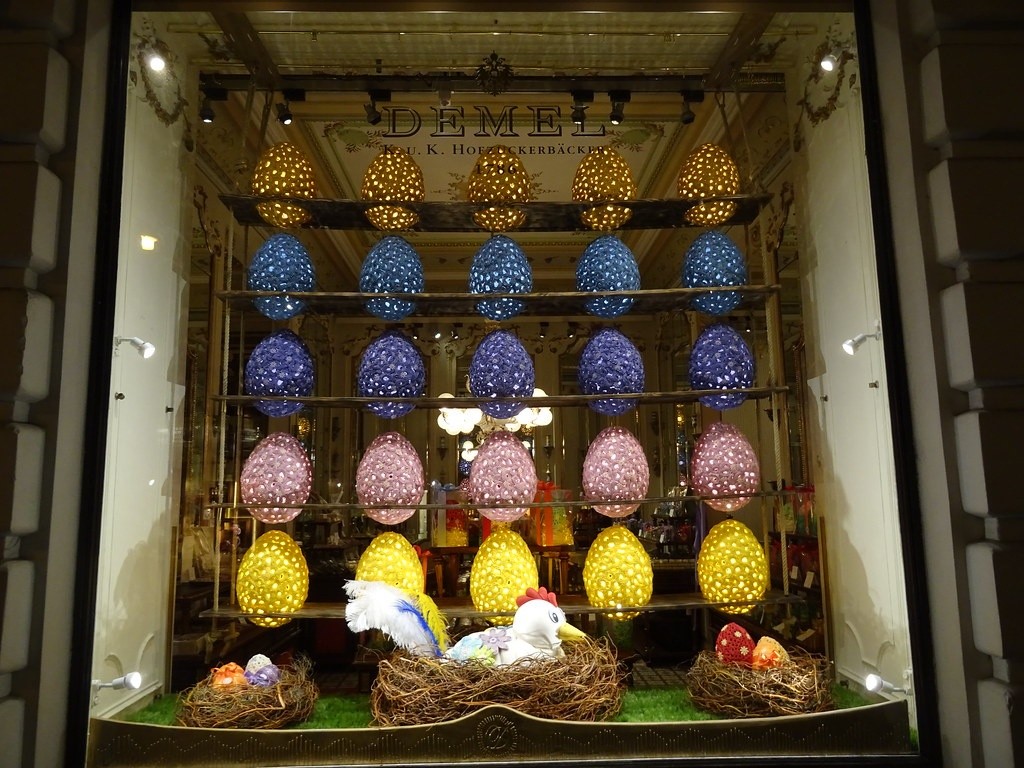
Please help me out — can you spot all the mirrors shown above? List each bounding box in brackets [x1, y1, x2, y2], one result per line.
[451, 351, 538, 488]
[348, 337, 433, 536]
[558, 338, 620, 515]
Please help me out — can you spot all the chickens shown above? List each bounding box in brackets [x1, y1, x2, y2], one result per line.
[344, 580, 586, 668]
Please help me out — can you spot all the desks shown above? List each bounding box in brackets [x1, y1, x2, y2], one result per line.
[430, 544, 575, 600]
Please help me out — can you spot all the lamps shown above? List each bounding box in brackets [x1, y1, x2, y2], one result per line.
[116, 335, 156, 357]
[94, 669, 144, 693]
[680, 100, 696, 125]
[198, 98, 217, 122]
[820, 42, 849, 72]
[608, 102, 626, 124]
[841, 320, 880, 356]
[275, 100, 293, 125]
[569, 103, 589, 124]
[865, 669, 912, 695]
[240, 144, 768, 626]
[363, 101, 381, 126]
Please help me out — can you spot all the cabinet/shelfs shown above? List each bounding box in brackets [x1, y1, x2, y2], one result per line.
[210, 193, 808, 656]
